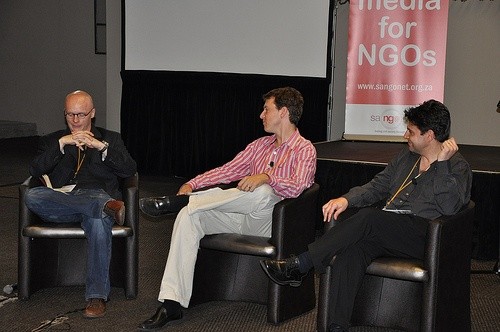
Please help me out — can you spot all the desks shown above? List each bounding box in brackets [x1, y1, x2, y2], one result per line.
[312, 138, 500, 276]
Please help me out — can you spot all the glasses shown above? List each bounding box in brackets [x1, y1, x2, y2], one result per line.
[64, 107, 95, 119]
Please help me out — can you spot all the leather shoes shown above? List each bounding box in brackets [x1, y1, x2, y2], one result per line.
[137, 306, 183, 331]
[138, 195, 179, 218]
[260, 254, 308, 286]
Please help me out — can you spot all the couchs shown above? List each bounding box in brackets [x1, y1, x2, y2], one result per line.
[189, 177, 320, 324]
[315, 197, 475, 332]
[18, 149, 139, 299]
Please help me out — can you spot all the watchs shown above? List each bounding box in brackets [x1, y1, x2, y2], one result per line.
[261, 99, 472, 332]
[98, 141, 110, 153]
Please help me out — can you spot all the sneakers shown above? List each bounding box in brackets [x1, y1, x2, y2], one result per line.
[83, 298, 107, 317]
[103, 200, 125, 226]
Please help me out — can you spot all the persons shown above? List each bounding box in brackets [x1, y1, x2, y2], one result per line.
[137, 86, 318, 330]
[23, 90, 138, 319]
